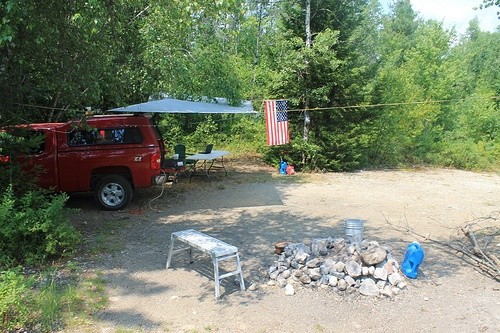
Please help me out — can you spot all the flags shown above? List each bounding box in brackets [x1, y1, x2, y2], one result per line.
[264, 99, 290, 146]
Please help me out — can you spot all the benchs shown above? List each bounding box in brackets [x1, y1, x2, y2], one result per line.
[166, 229, 245, 302]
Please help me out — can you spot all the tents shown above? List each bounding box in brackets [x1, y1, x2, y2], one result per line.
[106, 98, 259, 182]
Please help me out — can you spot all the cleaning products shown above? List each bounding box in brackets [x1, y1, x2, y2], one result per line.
[400, 243, 424, 280]
[286, 165, 295, 175]
[280, 162, 287, 174]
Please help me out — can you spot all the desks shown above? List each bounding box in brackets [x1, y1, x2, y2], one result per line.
[185, 150, 230, 184]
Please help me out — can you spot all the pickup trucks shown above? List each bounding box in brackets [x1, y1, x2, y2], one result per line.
[0, 116, 167, 210]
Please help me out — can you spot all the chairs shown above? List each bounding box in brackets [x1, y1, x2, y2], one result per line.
[174, 145, 195, 174]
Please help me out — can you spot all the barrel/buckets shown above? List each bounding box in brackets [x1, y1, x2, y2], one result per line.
[401, 241, 424, 279]
[280, 161, 287, 174]
[344, 219, 364, 241]
